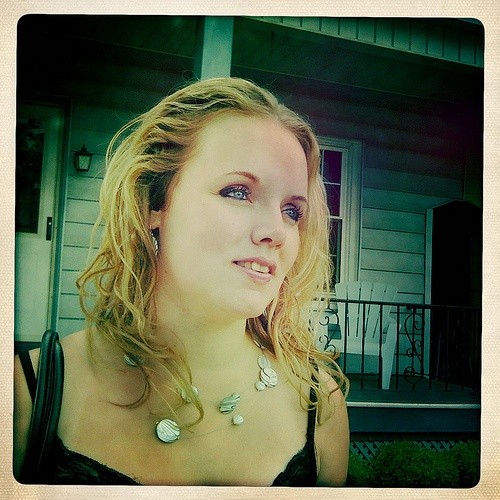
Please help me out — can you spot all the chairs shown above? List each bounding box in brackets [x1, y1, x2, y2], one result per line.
[313, 281, 402, 391]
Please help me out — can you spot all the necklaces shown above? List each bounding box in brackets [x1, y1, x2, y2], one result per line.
[122, 339, 277, 444]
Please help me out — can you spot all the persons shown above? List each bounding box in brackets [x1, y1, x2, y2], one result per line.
[14, 76, 350, 486]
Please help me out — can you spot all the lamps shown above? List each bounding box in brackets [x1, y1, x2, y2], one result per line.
[73, 143, 93, 171]
